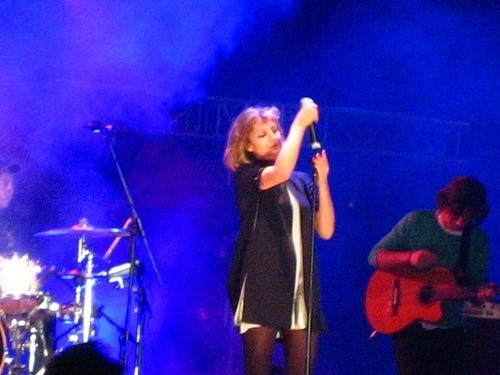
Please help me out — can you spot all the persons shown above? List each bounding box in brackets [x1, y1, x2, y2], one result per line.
[221, 99, 337, 375]
[0, 151, 38, 259]
[366, 176, 497, 375]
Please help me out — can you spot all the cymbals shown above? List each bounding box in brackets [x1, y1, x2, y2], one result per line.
[34, 226, 132, 238]
[59, 269, 83, 281]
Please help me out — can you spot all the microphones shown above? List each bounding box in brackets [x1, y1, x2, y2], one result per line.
[300, 97, 321, 151]
[86, 120, 118, 133]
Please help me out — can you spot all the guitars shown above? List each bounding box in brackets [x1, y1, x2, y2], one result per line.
[366, 267, 500, 334]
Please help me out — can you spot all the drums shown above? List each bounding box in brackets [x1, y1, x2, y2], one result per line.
[53, 303, 97, 356]
[0, 324, 7, 375]
[0, 254, 40, 316]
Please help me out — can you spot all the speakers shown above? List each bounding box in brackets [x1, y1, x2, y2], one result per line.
[460, 316, 499, 375]
